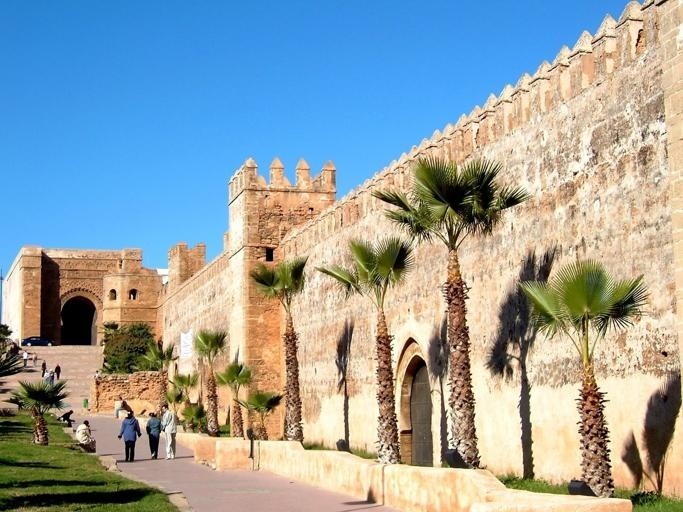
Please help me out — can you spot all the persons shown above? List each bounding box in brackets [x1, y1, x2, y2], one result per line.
[48, 369, 54, 384]
[60, 410, 75, 427]
[117, 410, 141, 460]
[76, 420, 95, 452]
[41, 359, 45, 376]
[54, 364, 59, 379]
[114, 397, 125, 418]
[43, 369, 48, 383]
[31, 352, 37, 366]
[22, 349, 28, 366]
[160, 404, 177, 459]
[145, 411, 162, 458]
[95, 370, 98, 376]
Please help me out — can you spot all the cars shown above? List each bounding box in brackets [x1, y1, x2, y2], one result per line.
[20, 335, 56, 347]
